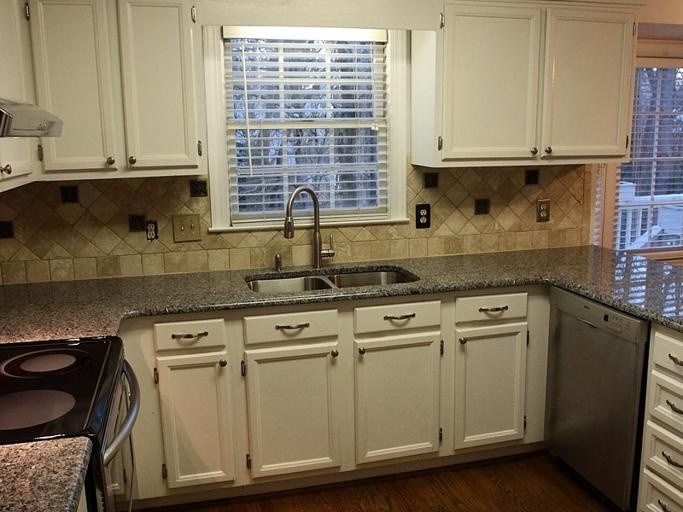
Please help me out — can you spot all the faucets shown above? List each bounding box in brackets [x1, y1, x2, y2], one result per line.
[283, 185, 335, 269]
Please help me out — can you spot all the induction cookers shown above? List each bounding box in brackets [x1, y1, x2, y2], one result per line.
[0, 336, 124, 443]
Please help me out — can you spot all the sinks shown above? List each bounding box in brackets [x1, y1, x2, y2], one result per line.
[326, 270, 416, 288]
[248, 278, 330, 293]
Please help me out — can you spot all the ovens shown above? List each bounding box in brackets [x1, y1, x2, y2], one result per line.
[85, 361, 142, 512]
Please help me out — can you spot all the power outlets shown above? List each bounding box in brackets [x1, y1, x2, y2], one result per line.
[413, 202, 432, 230]
[145, 221, 159, 241]
[535, 198, 550, 224]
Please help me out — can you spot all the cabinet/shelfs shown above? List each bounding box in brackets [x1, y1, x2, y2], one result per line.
[631, 323, 683, 512]
[118, 312, 239, 511]
[225, 281, 453, 499]
[0, 1, 36, 196]
[19, 0, 209, 184]
[436, 283, 550, 472]
[409, 2, 650, 168]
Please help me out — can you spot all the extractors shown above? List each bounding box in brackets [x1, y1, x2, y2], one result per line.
[1, 98, 64, 141]
